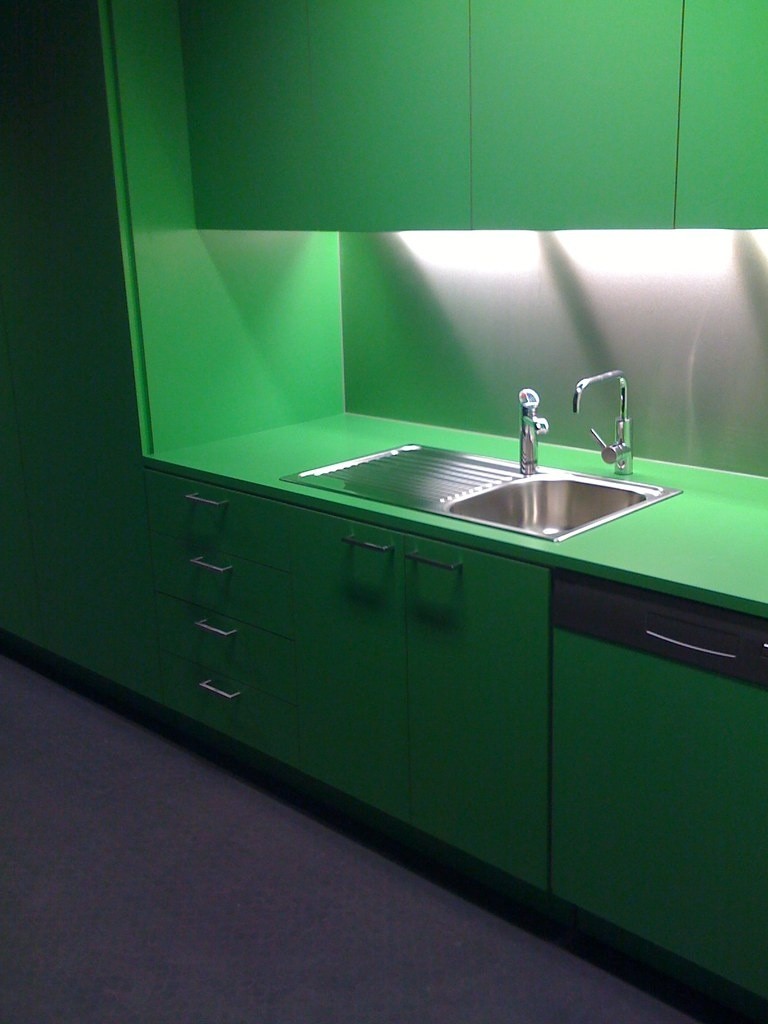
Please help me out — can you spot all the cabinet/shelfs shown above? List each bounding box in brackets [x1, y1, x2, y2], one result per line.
[1, 1, 768, 1024]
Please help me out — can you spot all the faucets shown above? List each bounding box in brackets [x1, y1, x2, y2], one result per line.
[572, 369, 634, 474]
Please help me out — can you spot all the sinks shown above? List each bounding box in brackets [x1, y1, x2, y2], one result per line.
[438, 473, 683, 543]
